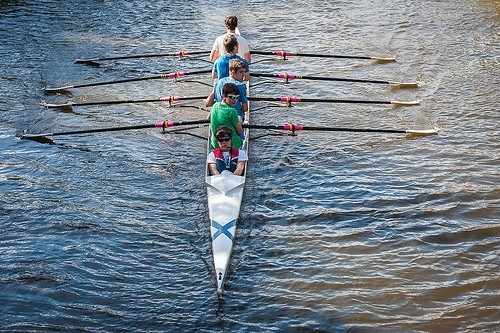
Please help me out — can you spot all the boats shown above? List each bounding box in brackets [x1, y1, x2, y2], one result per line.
[204, 27, 250, 298]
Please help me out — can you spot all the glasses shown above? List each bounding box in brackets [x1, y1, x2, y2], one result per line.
[218, 138, 231, 142]
[227, 96, 239, 99]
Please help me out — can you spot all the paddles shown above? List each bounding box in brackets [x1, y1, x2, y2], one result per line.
[75, 50, 211, 62]
[249, 72, 417, 85]
[45, 69, 212, 91]
[246, 97, 420, 105]
[22, 119, 211, 137]
[241, 123, 439, 135]
[45, 95, 209, 107]
[250, 50, 396, 60]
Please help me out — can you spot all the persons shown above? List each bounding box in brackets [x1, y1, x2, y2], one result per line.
[212, 35, 249, 82]
[207, 83, 242, 150]
[207, 125, 248, 175]
[205, 58, 248, 123]
[210, 15, 251, 64]
